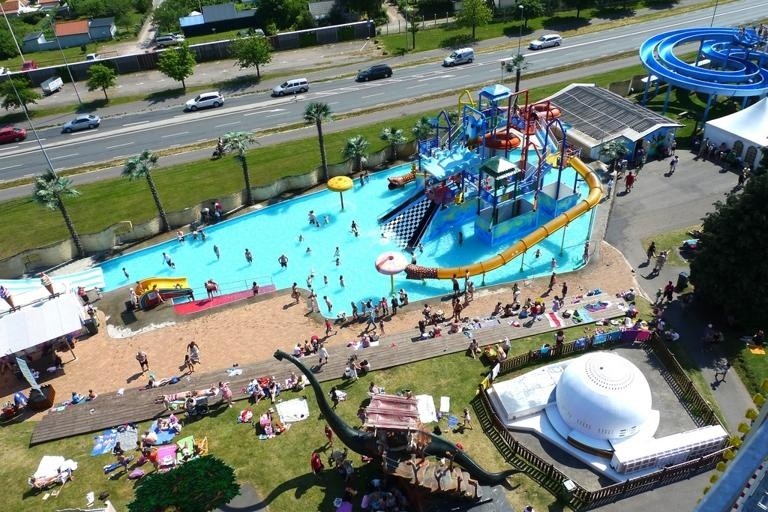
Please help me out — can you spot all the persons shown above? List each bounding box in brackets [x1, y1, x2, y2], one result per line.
[0, 135, 767, 511]
[738, 24, 767, 41]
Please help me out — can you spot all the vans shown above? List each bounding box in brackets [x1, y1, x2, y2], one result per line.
[528, 33, 562, 51]
[442, 46, 474, 68]
[272, 76, 310, 96]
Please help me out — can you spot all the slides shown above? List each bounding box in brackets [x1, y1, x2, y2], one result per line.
[404, 154, 604, 279]
[135, 277, 191, 311]
[639, 27, 768, 96]
[466, 105, 561, 149]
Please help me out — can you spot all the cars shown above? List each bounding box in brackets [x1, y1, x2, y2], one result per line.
[0, 125, 27, 143]
[61, 113, 101, 134]
[0, 31, 185, 76]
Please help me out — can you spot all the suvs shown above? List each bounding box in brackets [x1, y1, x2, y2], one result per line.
[184, 90, 224, 112]
[356, 62, 392, 82]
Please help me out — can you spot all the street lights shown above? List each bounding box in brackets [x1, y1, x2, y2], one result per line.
[45, 11, 82, 106]
[514, 2, 526, 95]
[3, 66, 59, 181]
